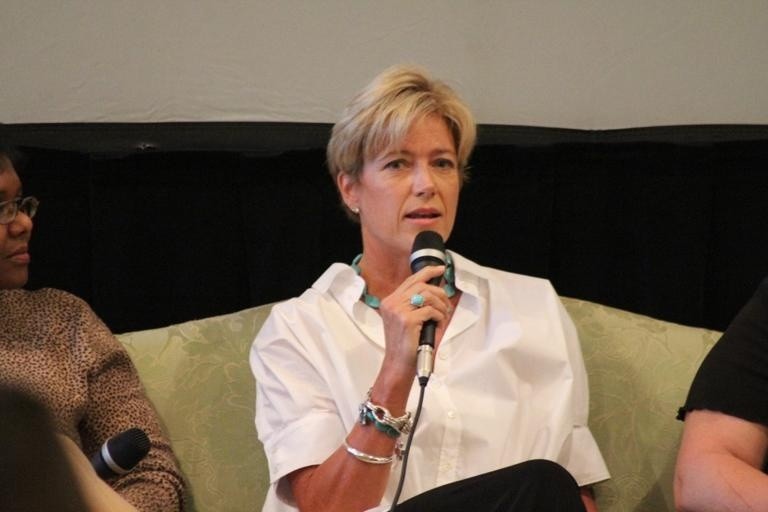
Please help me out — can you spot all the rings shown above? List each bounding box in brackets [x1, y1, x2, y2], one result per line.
[410, 292, 427, 309]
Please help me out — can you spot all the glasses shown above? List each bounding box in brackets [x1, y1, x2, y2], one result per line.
[0, 195, 40, 226]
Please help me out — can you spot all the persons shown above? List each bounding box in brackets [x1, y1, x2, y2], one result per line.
[242, 62, 616, 510]
[668, 267, 768, 512]
[0, 383, 144, 511]
[0, 131, 198, 511]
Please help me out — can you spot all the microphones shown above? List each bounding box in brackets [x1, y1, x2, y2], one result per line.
[408, 229, 446, 387]
[90, 427, 151, 480]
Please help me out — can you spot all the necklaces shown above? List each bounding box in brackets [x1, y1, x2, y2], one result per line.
[348, 251, 456, 308]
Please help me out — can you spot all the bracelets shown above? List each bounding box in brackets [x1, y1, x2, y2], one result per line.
[342, 433, 407, 465]
[357, 397, 414, 440]
